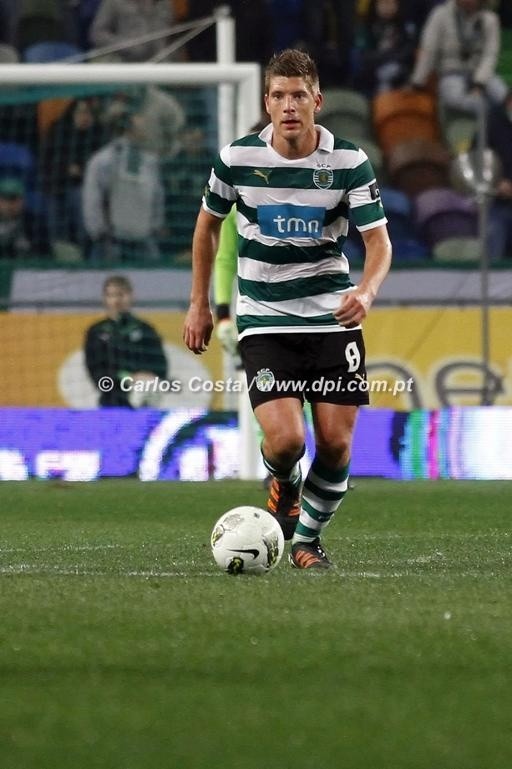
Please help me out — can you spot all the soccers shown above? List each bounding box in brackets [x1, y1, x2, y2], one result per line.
[209, 506, 285, 574]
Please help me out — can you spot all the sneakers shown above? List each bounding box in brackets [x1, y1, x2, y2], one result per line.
[266, 473, 302, 540]
[287, 537, 333, 569]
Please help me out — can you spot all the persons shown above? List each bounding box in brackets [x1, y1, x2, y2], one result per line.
[181, 47, 394, 576]
[83, 277, 169, 411]
[213, 116, 271, 354]
[1, 0, 512, 272]
